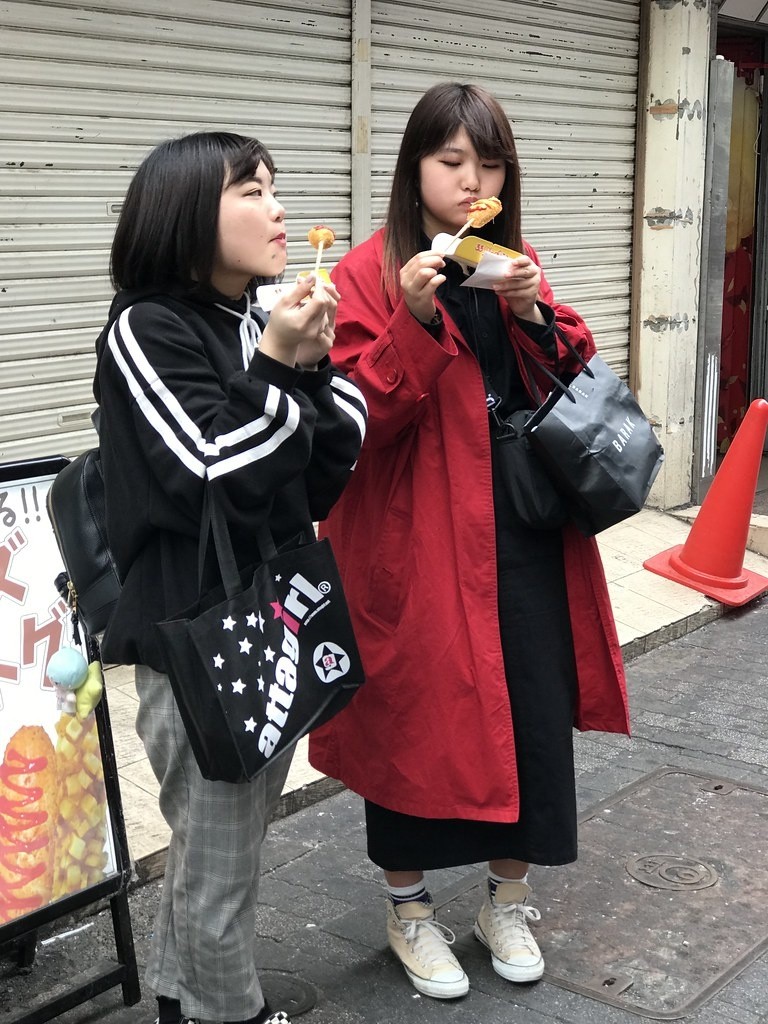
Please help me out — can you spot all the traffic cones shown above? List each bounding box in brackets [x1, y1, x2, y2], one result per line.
[642, 397, 768, 606]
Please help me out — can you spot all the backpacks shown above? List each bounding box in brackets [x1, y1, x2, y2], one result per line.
[44, 445, 124, 646]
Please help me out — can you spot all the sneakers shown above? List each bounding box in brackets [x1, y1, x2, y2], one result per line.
[473, 881, 545, 982]
[385, 890, 469, 999]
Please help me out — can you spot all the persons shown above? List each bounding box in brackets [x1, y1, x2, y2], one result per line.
[307, 80, 632, 1001]
[90, 133, 367, 1024]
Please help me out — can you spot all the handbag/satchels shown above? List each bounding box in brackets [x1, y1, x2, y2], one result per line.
[492, 410, 559, 529]
[150, 471, 366, 785]
[515, 322, 665, 541]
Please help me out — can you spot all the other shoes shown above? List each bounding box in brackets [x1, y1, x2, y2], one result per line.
[180, 1011, 293, 1024]
